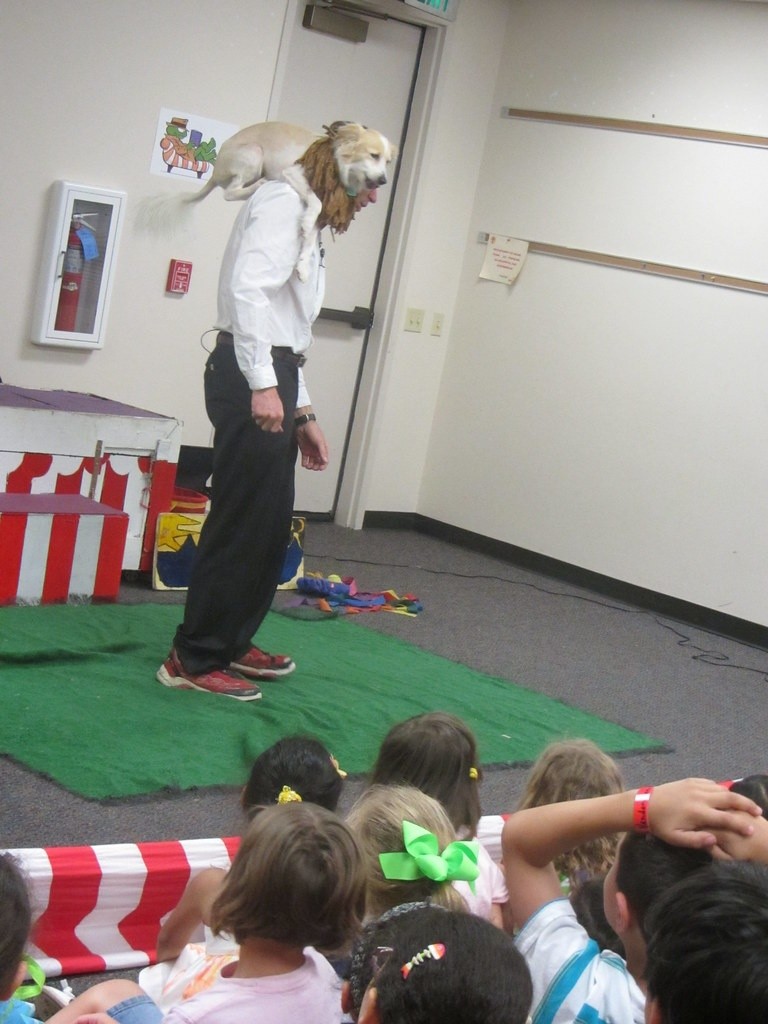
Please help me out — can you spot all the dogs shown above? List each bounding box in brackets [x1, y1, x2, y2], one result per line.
[180, 121, 397, 204]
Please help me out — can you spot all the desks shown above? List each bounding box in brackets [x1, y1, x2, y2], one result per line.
[0, 383, 184, 580]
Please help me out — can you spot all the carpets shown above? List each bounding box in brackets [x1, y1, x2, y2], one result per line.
[0, 594, 675, 803]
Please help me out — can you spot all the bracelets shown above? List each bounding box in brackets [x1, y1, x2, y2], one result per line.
[296, 414, 316, 425]
[633, 786, 652, 834]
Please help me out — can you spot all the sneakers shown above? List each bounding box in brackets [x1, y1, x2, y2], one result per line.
[228, 645, 296, 678]
[157, 646, 262, 702]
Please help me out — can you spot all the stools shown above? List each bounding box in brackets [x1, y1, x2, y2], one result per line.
[0, 491, 130, 604]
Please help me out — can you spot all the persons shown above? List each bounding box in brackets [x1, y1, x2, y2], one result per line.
[155, 121, 376, 700]
[1, 711, 768, 1024]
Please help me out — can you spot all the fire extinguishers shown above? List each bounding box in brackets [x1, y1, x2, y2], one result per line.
[54, 210, 99, 332]
